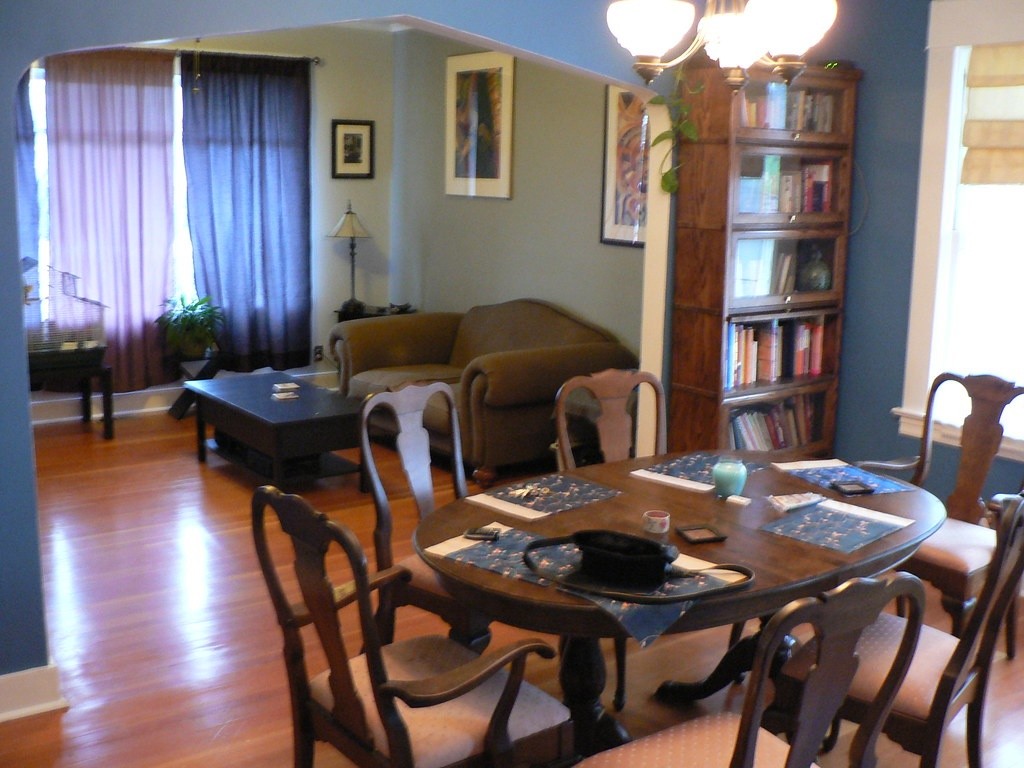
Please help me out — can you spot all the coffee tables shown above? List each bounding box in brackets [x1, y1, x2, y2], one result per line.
[183, 371, 374, 494]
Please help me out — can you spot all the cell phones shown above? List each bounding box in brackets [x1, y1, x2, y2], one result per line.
[465, 528, 500, 540]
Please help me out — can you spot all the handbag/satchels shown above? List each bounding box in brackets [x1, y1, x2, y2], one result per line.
[522, 527, 756, 604]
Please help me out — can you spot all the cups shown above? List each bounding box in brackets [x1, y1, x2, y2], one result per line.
[643, 510, 670, 534]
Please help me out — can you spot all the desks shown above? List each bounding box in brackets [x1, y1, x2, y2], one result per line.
[333, 306, 417, 323]
[412, 449, 946, 760]
[30, 363, 114, 439]
[158, 344, 236, 421]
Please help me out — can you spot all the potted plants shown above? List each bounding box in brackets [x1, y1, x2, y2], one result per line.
[153, 294, 228, 360]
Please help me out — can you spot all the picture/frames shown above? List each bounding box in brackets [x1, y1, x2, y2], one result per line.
[600, 83, 649, 249]
[331, 119, 375, 179]
[444, 51, 515, 198]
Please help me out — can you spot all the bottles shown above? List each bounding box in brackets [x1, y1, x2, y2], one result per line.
[205, 342, 219, 360]
[713, 457, 747, 497]
[799, 253, 831, 292]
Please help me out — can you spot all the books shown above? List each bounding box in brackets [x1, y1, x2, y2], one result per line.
[734, 238, 795, 297]
[724, 319, 826, 390]
[735, 81, 835, 132]
[725, 394, 818, 453]
[737, 152, 835, 212]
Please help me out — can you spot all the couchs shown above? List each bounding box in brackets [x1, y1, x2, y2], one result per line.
[324, 297, 638, 490]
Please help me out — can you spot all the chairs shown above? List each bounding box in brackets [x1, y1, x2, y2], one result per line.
[356, 380, 569, 658]
[776, 490, 1024, 768]
[850, 372, 1024, 660]
[251, 485, 578, 768]
[555, 368, 747, 712]
[566, 571, 928, 768]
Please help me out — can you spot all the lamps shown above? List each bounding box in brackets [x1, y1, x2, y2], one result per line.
[606, 0, 838, 86]
[325, 197, 373, 314]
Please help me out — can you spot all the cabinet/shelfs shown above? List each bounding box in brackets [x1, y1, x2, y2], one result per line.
[666, 54, 863, 459]
